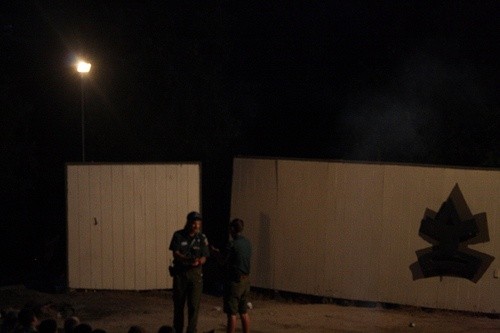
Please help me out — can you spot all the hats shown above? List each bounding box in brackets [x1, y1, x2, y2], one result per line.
[187, 211, 203, 221]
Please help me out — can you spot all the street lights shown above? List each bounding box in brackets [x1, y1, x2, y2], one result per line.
[76, 62, 92, 160]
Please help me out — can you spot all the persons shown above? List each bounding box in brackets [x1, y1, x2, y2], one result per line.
[0, 301, 107, 333]
[169, 211, 208, 333]
[223, 218, 251, 333]
[158, 326, 172, 333]
[128, 325, 146, 333]
